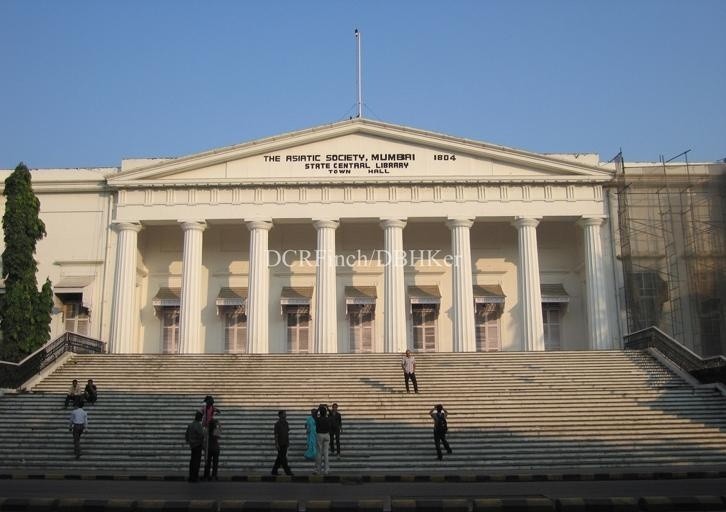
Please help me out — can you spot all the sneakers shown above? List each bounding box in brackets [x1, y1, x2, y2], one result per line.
[312, 452, 340, 476]
[272, 471, 295, 476]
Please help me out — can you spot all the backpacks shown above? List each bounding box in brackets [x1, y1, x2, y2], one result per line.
[436, 413, 447, 435]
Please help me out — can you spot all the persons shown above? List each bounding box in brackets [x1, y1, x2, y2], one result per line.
[328, 404, 342, 458]
[64, 378, 81, 409]
[310, 404, 334, 476]
[400, 349, 419, 394]
[68, 401, 88, 460]
[428, 404, 453, 461]
[204, 418, 221, 482]
[184, 411, 204, 483]
[83, 378, 98, 405]
[271, 409, 294, 476]
[304, 407, 318, 461]
[199, 395, 220, 431]
